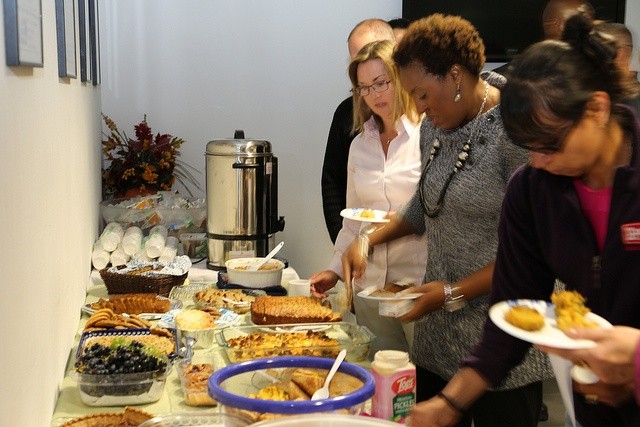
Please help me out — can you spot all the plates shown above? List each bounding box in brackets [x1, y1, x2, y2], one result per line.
[487, 298, 616, 351]
[355, 286, 424, 301]
[339, 208, 390, 225]
[246, 313, 338, 334]
[160, 309, 241, 331]
[83, 294, 182, 317]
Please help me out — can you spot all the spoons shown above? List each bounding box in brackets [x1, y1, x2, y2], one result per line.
[310, 349, 349, 403]
[245, 240, 285, 270]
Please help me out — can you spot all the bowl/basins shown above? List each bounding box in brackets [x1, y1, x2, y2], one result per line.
[224, 257, 285, 290]
[194, 288, 270, 313]
[207, 357, 376, 427]
[213, 321, 377, 363]
[100, 196, 208, 234]
[132, 412, 253, 427]
[69, 330, 175, 406]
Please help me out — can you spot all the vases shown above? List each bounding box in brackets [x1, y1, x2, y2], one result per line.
[116, 186, 158, 200]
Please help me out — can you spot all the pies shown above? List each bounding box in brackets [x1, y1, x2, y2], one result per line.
[87, 335, 174, 355]
[185, 362, 215, 389]
[175, 309, 216, 327]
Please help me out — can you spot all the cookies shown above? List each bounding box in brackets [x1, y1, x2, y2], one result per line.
[83, 308, 173, 336]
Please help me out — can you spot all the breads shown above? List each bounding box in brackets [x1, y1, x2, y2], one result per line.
[251, 297, 343, 325]
[235, 262, 282, 271]
[358, 207, 375, 218]
[256, 368, 342, 401]
[196, 287, 255, 310]
[91, 292, 171, 313]
[369, 280, 416, 298]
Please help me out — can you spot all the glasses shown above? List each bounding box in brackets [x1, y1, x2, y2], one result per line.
[354, 78, 392, 97]
[516, 145, 560, 155]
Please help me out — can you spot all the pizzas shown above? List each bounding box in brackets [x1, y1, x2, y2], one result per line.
[229, 329, 341, 357]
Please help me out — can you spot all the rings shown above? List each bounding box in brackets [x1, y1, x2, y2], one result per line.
[584, 394, 599, 401]
[573, 360, 585, 370]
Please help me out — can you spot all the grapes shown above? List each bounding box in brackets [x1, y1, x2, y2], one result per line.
[76, 340, 174, 395]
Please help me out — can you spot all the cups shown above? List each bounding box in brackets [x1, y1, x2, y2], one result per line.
[287, 280, 310, 299]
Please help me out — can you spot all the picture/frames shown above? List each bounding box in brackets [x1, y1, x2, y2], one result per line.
[79, 0, 91, 82]
[3, 0, 44, 68]
[92, 0, 101, 85]
[56, 0, 77, 79]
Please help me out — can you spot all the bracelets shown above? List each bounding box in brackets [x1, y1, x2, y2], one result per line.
[437, 390, 467, 417]
[442, 283, 452, 309]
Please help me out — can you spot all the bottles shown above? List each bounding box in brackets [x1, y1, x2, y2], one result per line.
[372, 350, 417, 420]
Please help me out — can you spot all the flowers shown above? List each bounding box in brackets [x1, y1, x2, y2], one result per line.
[102, 114, 204, 199]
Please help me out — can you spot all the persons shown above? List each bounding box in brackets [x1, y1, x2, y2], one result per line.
[320, 17, 413, 241]
[535, 324, 640, 404]
[409, 4, 640, 425]
[589, 21, 640, 116]
[346, 18, 396, 61]
[308, 39, 425, 359]
[341, 10, 546, 427]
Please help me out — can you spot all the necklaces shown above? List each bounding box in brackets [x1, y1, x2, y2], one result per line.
[383, 133, 395, 144]
[417, 78, 491, 218]
